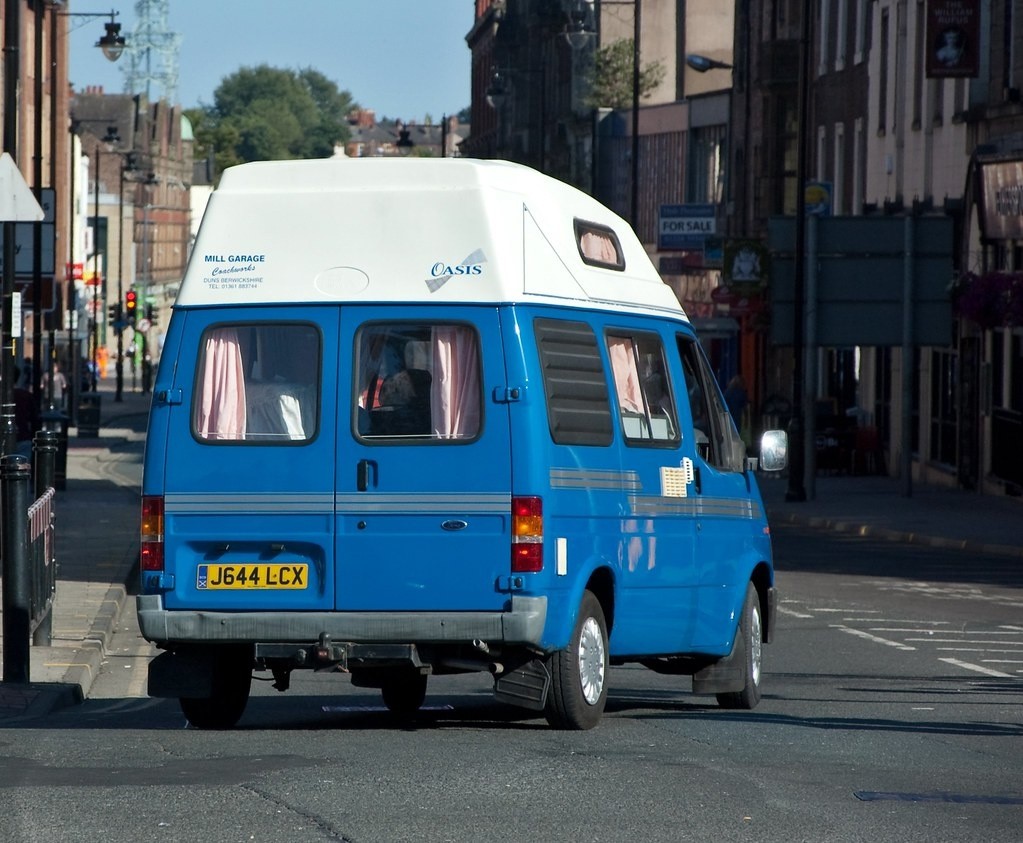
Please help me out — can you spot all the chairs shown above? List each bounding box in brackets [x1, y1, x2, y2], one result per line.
[370, 370, 430, 433]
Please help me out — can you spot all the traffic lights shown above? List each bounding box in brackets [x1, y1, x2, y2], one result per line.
[109, 304, 118, 328]
[127, 292, 138, 323]
[148, 303, 160, 327]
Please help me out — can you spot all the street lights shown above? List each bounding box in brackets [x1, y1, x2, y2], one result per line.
[396, 113, 448, 158]
[68, 115, 156, 403]
[559, 0, 641, 237]
[482, 66, 546, 172]
[48, 7, 131, 409]
[687, 54, 768, 448]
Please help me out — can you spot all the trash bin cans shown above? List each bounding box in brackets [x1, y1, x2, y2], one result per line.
[76, 392, 101, 439]
[30, 411, 70, 493]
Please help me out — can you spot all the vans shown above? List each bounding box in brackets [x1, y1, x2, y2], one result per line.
[135, 143, 789, 730]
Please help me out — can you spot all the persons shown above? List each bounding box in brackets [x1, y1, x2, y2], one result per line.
[14, 364, 38, 442]
[40, 360, 68, 414]
